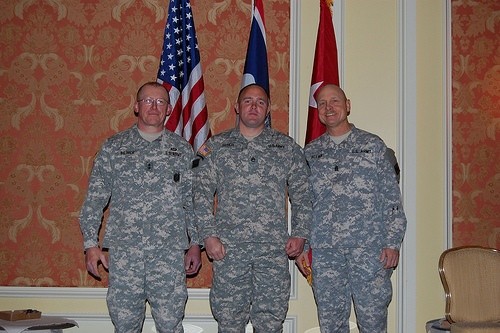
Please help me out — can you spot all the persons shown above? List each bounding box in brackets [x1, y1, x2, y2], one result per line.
[295, 84, 407, 333]
[191, 84, 315, 333]
[79, 83, 205, 333]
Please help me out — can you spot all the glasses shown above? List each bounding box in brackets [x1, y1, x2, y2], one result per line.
[136, 97, 170, 106]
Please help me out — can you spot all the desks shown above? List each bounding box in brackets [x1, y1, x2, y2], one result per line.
[1, 316, 77, 333]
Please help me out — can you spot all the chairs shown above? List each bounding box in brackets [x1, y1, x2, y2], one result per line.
[438, 245, 500, 332]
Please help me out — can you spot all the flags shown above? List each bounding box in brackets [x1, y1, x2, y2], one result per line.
[304, 0, 339, 288]
[157, 0, 212, 153]
[235, 0, 272, 129]
